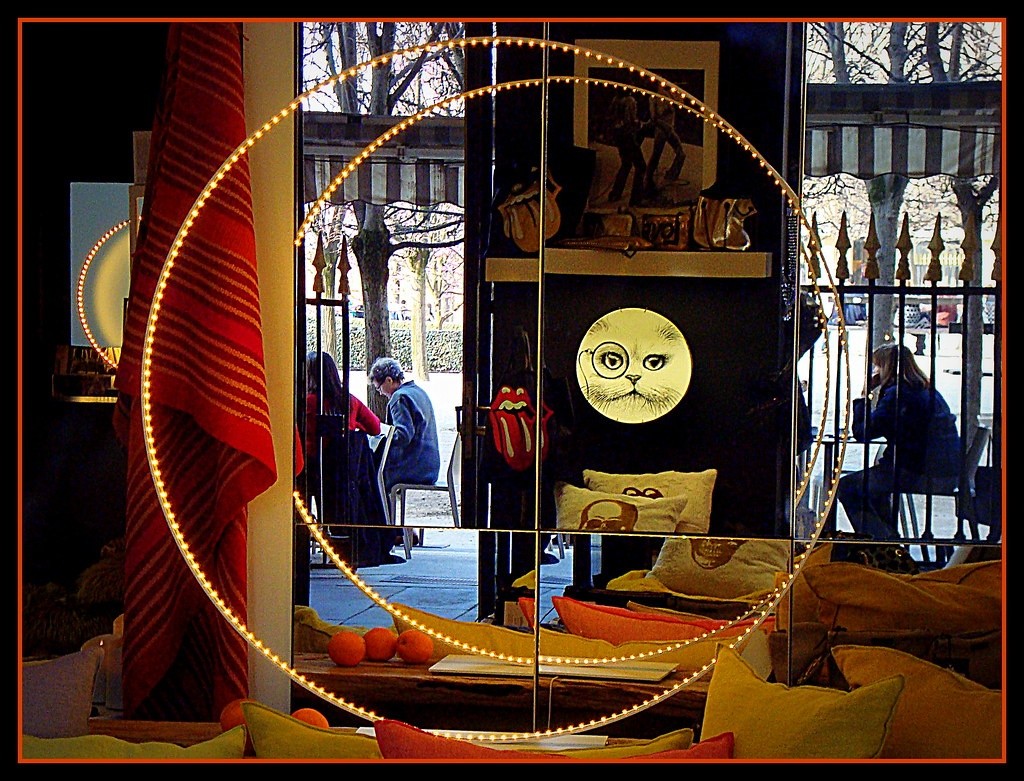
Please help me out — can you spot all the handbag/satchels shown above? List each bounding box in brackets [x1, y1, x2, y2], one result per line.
[818, 530, 919, 575]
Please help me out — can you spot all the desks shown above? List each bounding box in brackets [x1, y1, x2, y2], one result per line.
[813, 433, 891, 533]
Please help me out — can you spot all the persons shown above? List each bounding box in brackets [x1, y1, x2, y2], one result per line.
[369, 357, 440, 545]
[304, 351, 381, 493]
[836, 343, 964, 538]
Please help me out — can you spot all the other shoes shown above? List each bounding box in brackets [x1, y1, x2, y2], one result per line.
[395, 531, 419, 546]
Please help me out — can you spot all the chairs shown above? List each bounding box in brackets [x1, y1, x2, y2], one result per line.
[892, 424, 992, 559]
[327, 427, 396, 572]
[388, 429, 461, 562]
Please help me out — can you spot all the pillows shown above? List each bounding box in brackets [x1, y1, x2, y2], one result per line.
[22, 467, 1002, 761]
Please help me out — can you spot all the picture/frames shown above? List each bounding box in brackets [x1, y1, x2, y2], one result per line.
[574, 37, 720, 220]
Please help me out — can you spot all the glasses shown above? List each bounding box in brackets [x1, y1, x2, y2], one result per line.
[376, 379, 386, 392]
